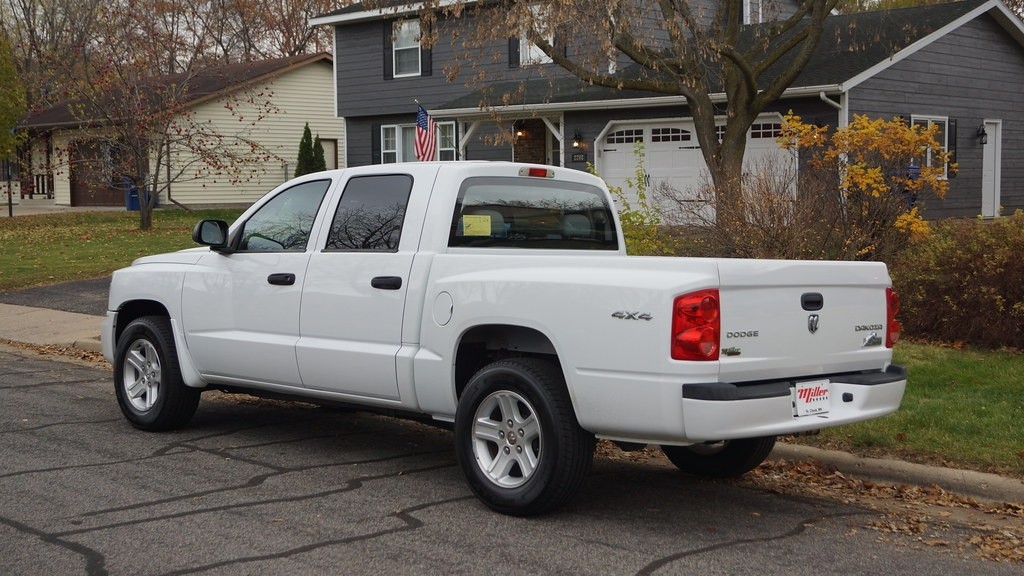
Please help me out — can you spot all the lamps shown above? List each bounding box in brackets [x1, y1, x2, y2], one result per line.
[54, 144, 60, 154]
[977, 124, 988, 144]
[570, 129, 582, 148]
[516, 124, 525, 141]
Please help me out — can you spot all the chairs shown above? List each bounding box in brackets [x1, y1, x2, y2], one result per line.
[559, 213, 592, 240]
[469, 210, 505, 238]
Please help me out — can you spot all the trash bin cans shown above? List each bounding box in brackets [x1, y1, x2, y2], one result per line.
[121, 174, 148, 211]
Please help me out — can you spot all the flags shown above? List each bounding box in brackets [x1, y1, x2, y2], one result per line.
[414, 106, 435, 161]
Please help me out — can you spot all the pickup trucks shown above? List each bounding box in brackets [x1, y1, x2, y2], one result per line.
[99, 158, 910, 520]
